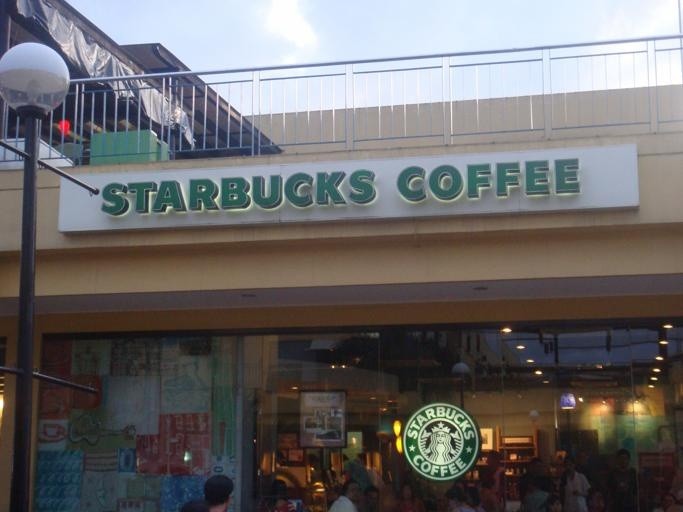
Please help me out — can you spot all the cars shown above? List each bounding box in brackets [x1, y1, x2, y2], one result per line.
[272, 451, 313, 494]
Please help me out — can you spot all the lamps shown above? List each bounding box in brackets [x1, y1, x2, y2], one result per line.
[558, 367, 576, 410]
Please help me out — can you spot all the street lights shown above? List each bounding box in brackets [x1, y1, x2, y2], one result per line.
[0, 37, 101, 511]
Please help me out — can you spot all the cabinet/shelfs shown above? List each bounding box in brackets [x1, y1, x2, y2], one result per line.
[496, 436, 538, 471]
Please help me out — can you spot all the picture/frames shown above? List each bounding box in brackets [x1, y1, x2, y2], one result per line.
[299, 390, 347, 449]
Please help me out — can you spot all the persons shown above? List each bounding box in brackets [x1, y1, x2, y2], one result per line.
[179, 500, 211, 511]
[203, 476, 234, 512]
[328, 448, 683, 512]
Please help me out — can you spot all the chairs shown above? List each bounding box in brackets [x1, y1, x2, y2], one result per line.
[266, 461, 578, 511]
